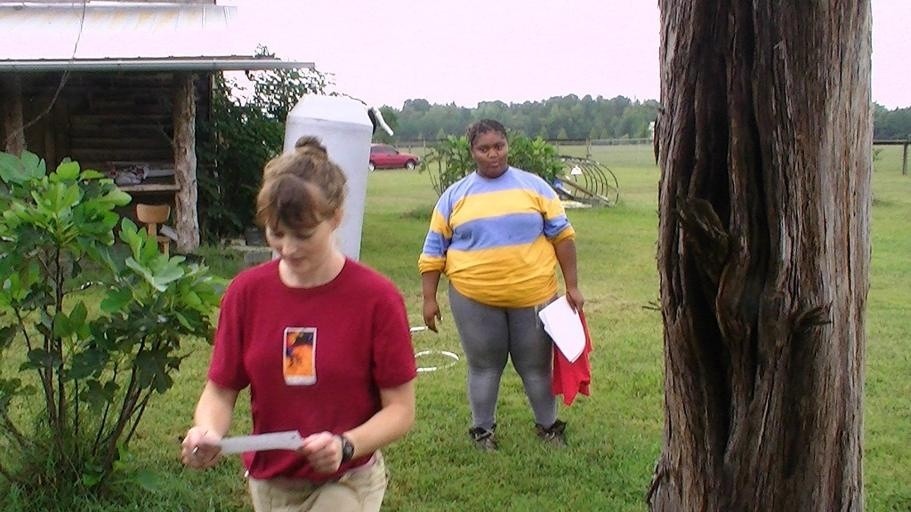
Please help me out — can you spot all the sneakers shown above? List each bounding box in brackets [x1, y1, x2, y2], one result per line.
[535, 420, 566, 449]
[469, 424, 497, 450]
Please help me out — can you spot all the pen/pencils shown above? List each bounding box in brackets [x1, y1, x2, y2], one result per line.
[191, 429, 209, 456]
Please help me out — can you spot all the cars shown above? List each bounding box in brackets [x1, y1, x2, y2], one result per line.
[369, 143, 420, 171]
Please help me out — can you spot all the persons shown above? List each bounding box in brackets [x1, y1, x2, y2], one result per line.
[417, 119, 585, 452]
[181, 135, 417, 512]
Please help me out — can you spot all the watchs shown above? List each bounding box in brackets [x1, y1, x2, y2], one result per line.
[341, 435, 354, 466]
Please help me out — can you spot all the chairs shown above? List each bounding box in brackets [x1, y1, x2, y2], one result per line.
[134, 202, 173, 260]
